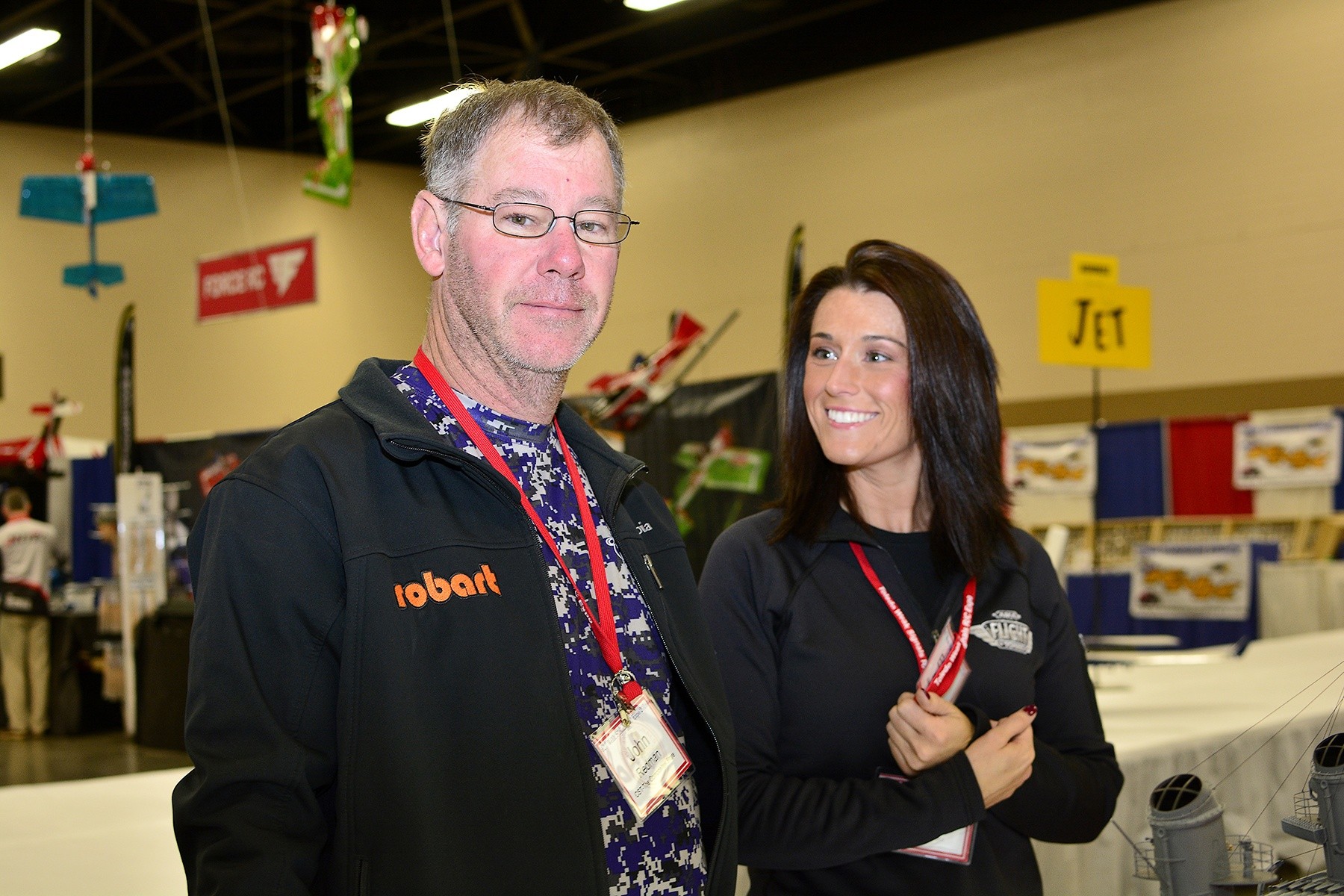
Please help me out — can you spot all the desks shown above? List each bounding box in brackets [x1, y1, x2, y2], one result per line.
[135, 612, 193, 750]
[1025, 628, 1344, 896]
[1065, 561, 1344, 650]
[48, 614, 103, 736]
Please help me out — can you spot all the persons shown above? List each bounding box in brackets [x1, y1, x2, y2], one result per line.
[1, 487, 69, 742]
[171, 78, 742, 893]
[700, 234, 1123, 896]
[90, 504, 130, 739]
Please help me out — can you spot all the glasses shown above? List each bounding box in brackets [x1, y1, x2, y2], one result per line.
[435, 197, 640, 245]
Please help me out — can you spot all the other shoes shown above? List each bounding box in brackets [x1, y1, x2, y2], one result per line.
[0, 729, 44, 742]
